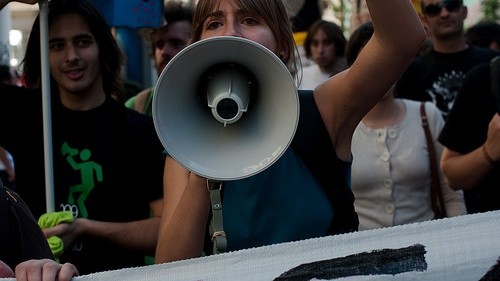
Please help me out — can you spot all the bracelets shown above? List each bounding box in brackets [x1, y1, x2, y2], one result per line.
[483, 144, 495, 165]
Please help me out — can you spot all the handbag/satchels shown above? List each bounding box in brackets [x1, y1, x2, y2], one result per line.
[0, 182, 55, 262]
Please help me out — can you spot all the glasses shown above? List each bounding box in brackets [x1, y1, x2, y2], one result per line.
[424, 0, 463, 18]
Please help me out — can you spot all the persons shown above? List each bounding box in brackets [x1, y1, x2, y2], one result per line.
[0, 0, 500, 281]
